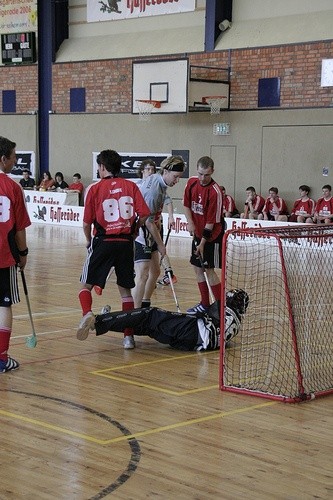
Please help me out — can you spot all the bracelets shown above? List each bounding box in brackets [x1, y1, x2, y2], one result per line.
[202, 228, 211, 241]
[19, 247, 28, 256]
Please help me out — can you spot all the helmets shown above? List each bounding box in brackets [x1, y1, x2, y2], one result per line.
[225, 289, 249, 312]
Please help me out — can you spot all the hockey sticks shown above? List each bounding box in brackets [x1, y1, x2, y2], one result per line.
[194, 236, 215, 303]
[160, 230, 171, 265]
[21, 271, 37, 348]
[94, 267, 115, 295]
[167, 272, 181, 313]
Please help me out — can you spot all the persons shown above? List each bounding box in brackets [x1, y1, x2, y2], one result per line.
[91, 289, 250, 352]
[306, 185, 333, 223]
[258, 188, 289, 220]
[220, 186, 239, 218]
[37, 172, 54, 190]
[181, 156, 227, 314]
[134, 155, 186, 311]
[75, 149, 167, 349]
[49, 172, 68, 192]
[241, 186, 264, 219]
[0, 136, 32, 372]
[278, 185, 315, 221]
[65, 173, 83, 205]
[19, 171, 35, 190]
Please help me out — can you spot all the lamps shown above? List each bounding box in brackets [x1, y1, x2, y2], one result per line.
[219, 20, 232, 31]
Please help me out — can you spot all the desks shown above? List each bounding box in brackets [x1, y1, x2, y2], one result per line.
[23, 190, 79, 206]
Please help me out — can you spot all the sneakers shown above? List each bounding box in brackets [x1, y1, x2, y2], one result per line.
[0, 356, 19, 374]
[90, 314, 99, 331]
[101, 305, 111, 314]
[77, 312, 95, 341]
[156, 275, 177, 286]
[185, 303, 210, 314]
[123, 335, 135, 349]
